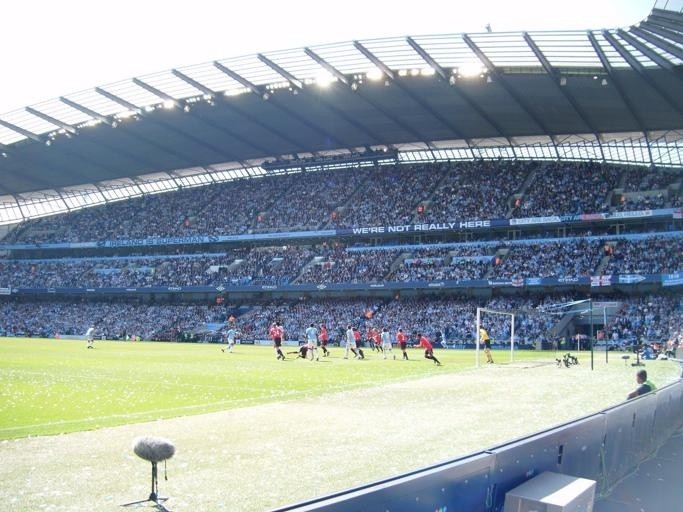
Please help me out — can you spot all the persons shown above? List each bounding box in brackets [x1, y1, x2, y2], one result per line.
[626, 368, 658, 400]
[1, 158, 683, 367]
